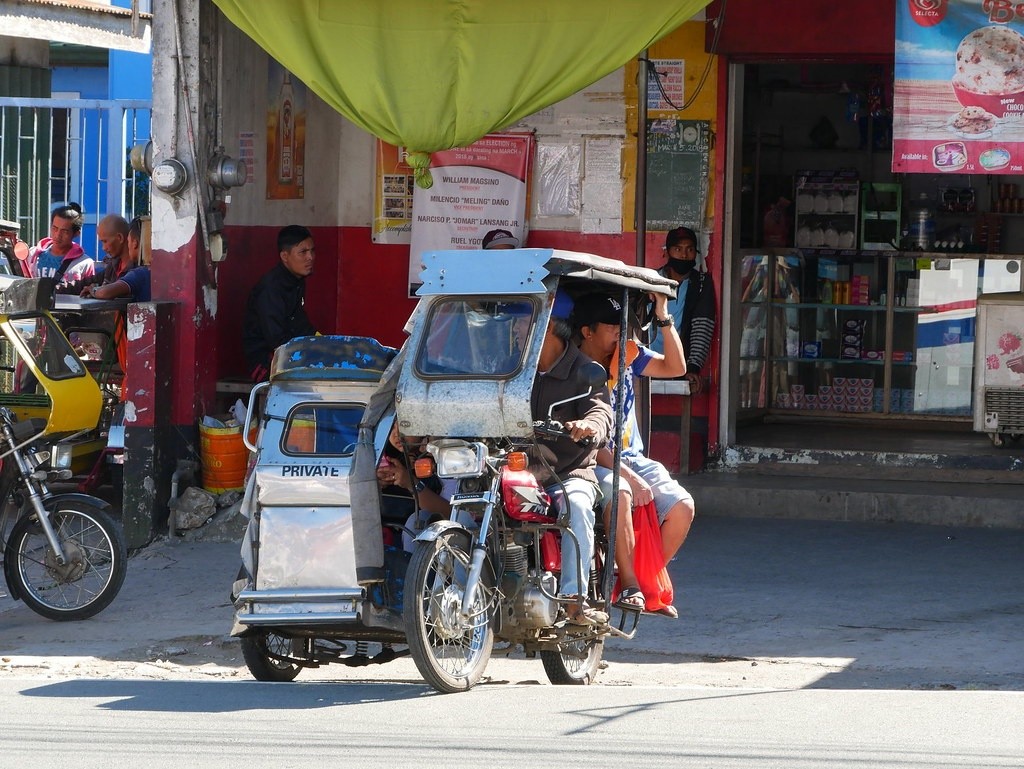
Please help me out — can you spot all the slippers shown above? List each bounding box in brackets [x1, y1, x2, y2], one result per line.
[657, 604, 679, 618]
[615, 588, 645, 611]
[565, 607, 609, 624]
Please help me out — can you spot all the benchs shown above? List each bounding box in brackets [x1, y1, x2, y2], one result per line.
[217, 380, 267, 418]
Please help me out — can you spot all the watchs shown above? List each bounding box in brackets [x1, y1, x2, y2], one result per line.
[94, 287, 100, 298]
[410, 481, 425, 494]
[657, 314, 675, 327]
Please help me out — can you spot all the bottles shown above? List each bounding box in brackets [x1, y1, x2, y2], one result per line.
[821, 278, 833, 304]
[907, 192, 935, 251]
[880, 290, 887, 306]
[901, 293, 906, 306]
[894, 293, 900, 306]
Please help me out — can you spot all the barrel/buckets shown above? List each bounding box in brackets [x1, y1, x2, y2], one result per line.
[198, 413, 258, 493]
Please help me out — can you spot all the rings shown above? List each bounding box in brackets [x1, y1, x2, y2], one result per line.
[393, 476, 395, 481]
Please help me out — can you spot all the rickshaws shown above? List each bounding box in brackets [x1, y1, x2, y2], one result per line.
[229, 241, 681, 694]
[0, 272, 136, 624]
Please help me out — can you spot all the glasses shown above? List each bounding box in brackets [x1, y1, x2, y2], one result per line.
[134, 215, 140, 230]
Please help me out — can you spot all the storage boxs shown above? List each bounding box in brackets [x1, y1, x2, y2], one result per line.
[740, 250, 1024, 424]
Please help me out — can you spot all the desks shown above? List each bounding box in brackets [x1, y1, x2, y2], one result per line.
[53, 293, 121, 322]
[651, 380, 699, 475]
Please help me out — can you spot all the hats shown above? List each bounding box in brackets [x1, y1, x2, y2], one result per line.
[497, 288, 574, 319]
[573, 294, 623, 325]
[666, 227, 698, 251]
[482, 229, 519, 249]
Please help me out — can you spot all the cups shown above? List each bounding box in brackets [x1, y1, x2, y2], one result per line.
[991, 183, 1024, 213]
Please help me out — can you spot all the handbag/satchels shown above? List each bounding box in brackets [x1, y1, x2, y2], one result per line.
[611, 499, 674, 611]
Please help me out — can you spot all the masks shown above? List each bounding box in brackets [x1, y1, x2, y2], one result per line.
[668, 257, 696, 275]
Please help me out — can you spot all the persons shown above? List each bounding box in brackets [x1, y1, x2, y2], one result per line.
[243, 224, 322, 382]
[443, 228, 519, 370]
[575, 290, 695, 618]
[22, 208, 151, 349]
[378, 424, 458, 612]
[647, 227, 716, 396]
[434, 293, 609, 624]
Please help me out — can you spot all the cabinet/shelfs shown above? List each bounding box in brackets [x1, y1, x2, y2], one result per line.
[744, 124, 904, 251]
